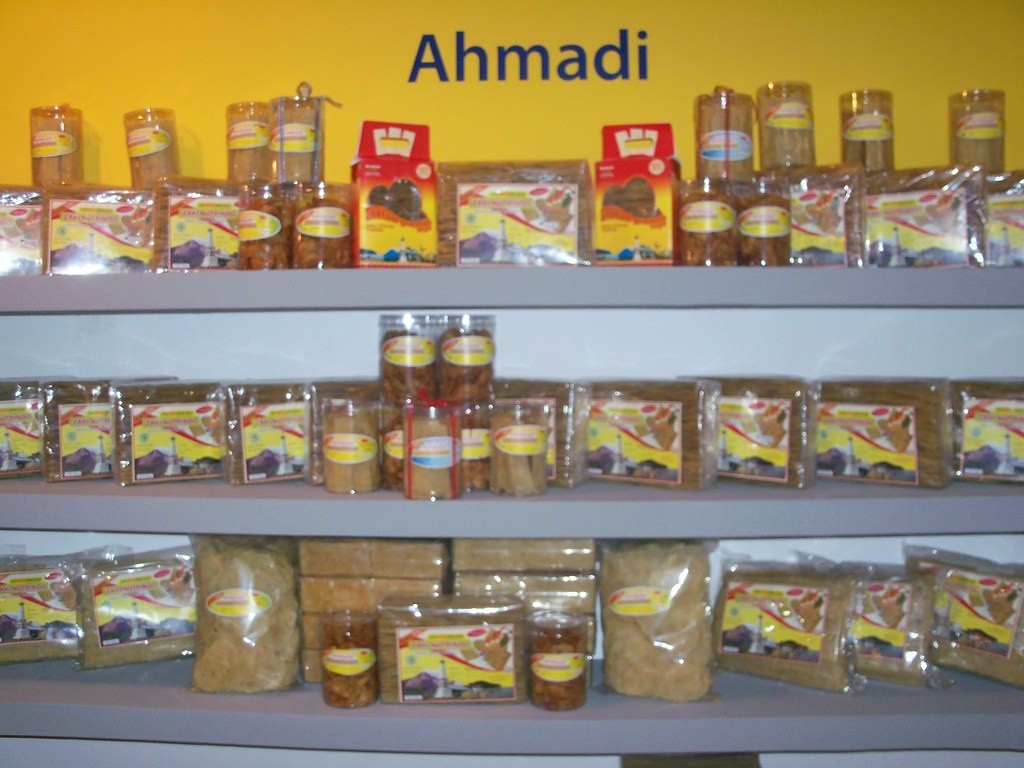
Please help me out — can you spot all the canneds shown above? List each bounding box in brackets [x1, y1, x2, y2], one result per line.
[737, 177, 792, 266]
[319, 315, 548, 500]
[678, 180, 736, 267]
[239, 181, 291, 268]
[271, 97, 324, 183]
[226, 101, 272, 180]
[948, 89, 1004, 174]
[293, 184, 351, 269]
[694, 94, 754, 181]
[124, 107, 181, 187]
[526, 611, 586, 710]
[840, 89, 894, 173]
[30, 106, 83, 186]
[758, 81, 816, 165]
[321, 610, 379, 708]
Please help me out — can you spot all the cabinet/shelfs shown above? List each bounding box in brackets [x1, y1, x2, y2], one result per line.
[0, 258, 1024, 756]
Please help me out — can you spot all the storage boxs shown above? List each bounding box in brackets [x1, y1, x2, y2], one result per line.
[588, 122, 682, 266]
[348, 121, 438, 268]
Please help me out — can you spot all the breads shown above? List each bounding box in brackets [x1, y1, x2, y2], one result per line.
[0, 379, 1024, 702]
[0, 158, 1024, 268]
